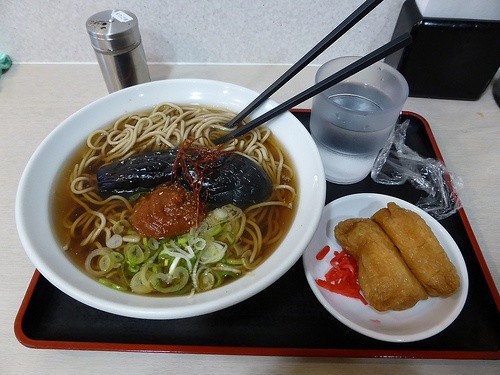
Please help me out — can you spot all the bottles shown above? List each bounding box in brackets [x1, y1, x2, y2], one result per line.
[86, 8, 150, 94]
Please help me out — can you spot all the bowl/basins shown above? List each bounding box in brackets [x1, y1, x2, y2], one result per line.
[15, 78, 326, 320]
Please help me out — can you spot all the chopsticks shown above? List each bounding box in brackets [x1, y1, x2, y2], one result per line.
[208, 0, 411, 145]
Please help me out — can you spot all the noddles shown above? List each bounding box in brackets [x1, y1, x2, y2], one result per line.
[48, 103, 296, 297]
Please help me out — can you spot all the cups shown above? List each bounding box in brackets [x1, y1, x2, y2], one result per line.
[309, 55, 409, 184]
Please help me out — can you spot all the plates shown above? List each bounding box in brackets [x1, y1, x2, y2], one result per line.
[302, 193, 468, 342]
